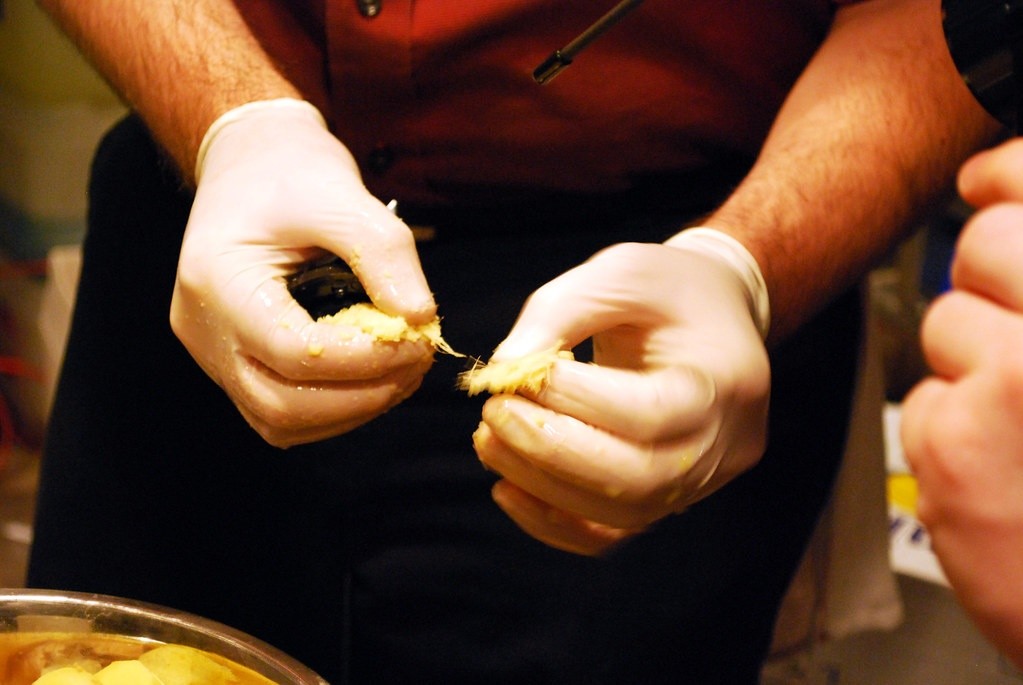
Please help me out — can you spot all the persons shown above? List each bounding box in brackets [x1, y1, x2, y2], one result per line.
[898, 137, 1023, 677]
[31, 0, 1023, 685]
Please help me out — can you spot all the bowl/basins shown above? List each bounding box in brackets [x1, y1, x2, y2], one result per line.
[1, 586, 326, 685]
